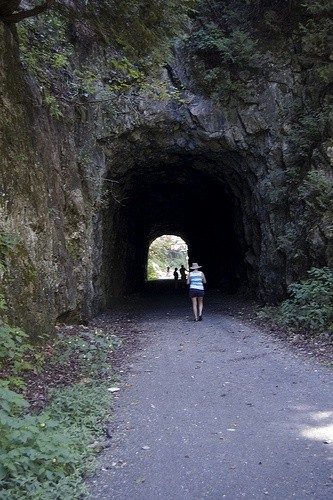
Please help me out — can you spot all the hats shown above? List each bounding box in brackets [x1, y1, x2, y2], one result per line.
[190, 263, 202, 269]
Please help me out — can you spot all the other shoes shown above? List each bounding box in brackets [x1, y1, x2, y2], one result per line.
[198, 316, 202, 321]
[194, 318, 198, 322]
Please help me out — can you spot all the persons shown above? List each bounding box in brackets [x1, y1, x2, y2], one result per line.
[187, 263, 206, 321]
[167, 265, 188, 288]
